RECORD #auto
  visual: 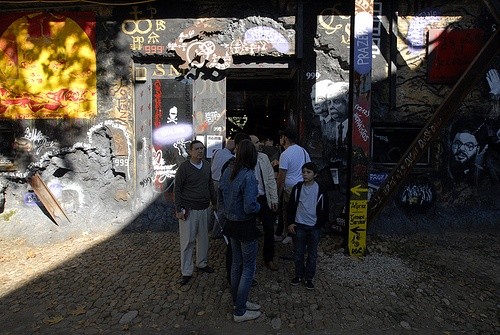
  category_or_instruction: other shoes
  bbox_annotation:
[281,236,293,245]
[196,265,213,273]
[304,279,314,290]
[181,276,190,285]
[233,301,262,311]
[290,276,304,285]
[273,235,283,241]
[233,310,261,323]
[266,261,278,270]
[251,279,257,286]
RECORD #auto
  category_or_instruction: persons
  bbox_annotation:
[310,79,336,149]
[326,81,348,145]
[219,139,261,322]
[209,135,279,285]
[274,128,311,244]
[173,141,216,284]
[285,163,326,290]
